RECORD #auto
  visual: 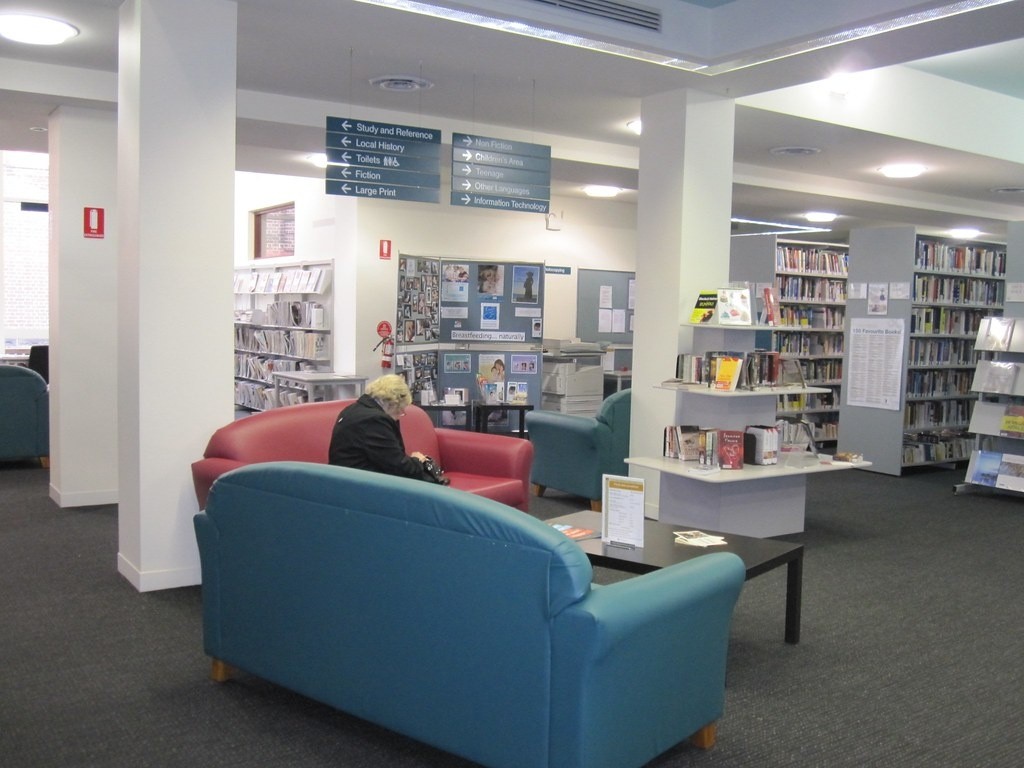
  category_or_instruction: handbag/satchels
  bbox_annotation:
[420,455,451,486]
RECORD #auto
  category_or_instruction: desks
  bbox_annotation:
[474,399,535,439]
[271,371,369,408]
[605,370,631,391]
[419,401,473,432]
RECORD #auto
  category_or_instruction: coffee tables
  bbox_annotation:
[543,509,806,646]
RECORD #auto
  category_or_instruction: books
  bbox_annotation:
[972,450,1003,486]
[1000,395,1024,439]
[833,451,863,462]
[902,240,1006,465]
[775,246,848,456]
[662,286,781,470]
[549,523,601,541]
[995,453,1024,493]
[235,270,328,409]
[983,362,1017,394]
[983,318,1014,350]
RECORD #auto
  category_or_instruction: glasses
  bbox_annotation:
[400,408,407,419]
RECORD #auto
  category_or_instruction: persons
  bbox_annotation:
[397,258,440,341]
[447,361,469,370]
[509,386,515,394]
[521,363,534,370]
[489,392,496,401]
[444,265,468,281]
[490,360,505,382]
[330,374,438,482]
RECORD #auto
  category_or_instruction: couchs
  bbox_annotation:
[0,364,50,468]
[191,398,534,515]
[523,386,631,512]
[193,460,746,768]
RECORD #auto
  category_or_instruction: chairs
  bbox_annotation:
[28,345,49,383]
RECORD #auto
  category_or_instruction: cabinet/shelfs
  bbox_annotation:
[724,233,849,447]
[837,225,1006,477]
[622,323,873,539]
[234,258,336,415]
